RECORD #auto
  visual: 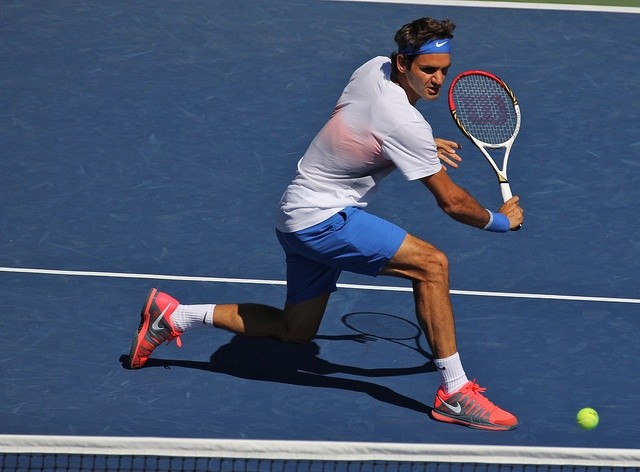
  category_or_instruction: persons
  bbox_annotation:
[130,16,523,431]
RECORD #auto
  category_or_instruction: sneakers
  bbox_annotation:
[431,378,518,431]
[128,287,184,369]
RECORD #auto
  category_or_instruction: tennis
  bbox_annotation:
[575,407,600,430]
